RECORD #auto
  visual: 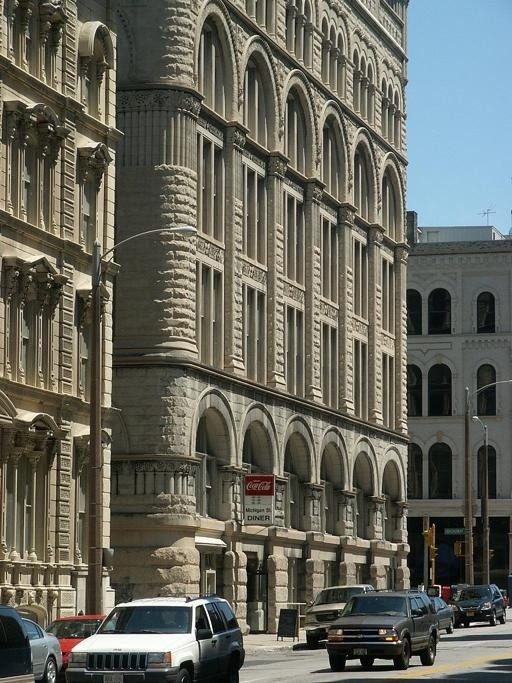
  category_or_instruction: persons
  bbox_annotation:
[69,623,77,634]
[174,611,187,627]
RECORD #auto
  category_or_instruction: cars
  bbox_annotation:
[0,606,111,683]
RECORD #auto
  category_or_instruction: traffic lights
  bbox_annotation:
[429,546,439,559]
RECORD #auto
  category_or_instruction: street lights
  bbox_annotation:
[85,227,200,606]
[472,414,491,585]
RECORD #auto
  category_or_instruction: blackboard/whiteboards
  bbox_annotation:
[278,609,298,637]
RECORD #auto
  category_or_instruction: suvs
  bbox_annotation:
[305,585,455,669]
[454,584,506,626]
[65,594,245,682]
